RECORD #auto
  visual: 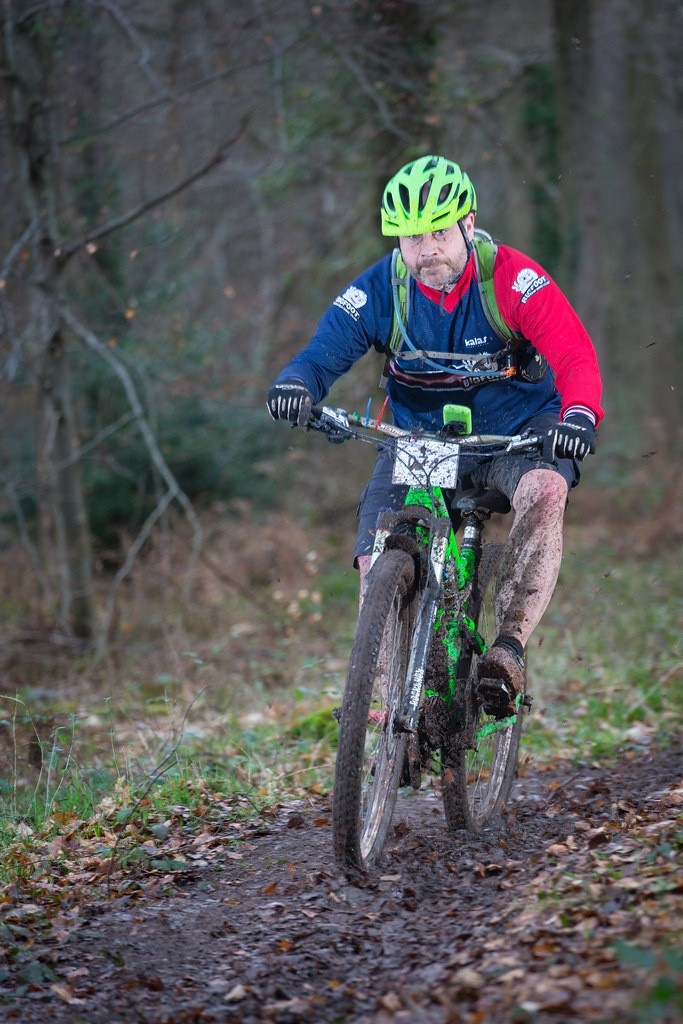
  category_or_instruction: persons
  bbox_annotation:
[265,156,604,784]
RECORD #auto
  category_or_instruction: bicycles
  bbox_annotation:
[289,402,599,884]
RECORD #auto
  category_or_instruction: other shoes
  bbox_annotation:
[370,734,431,777]
[477,644,524,716]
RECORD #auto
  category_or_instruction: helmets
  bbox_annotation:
[381,155,477,237]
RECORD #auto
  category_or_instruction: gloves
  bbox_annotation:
[266,380,315,433]
[543,412,596,464]
